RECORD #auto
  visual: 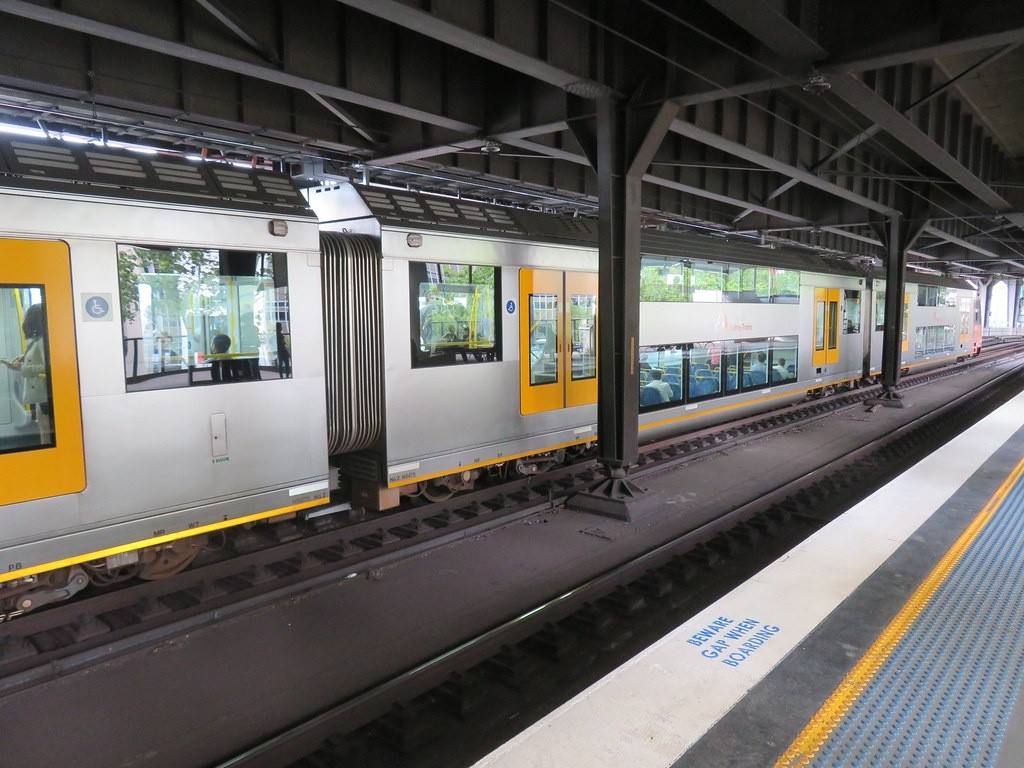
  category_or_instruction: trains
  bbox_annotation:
[0,97,983,590]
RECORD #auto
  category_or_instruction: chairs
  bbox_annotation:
[639,362,795,406]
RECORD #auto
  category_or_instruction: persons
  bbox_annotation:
[442,326,483,360]
[640,354,652,372]
[420,286,474,351]
[646,371,673,403]
[707,343,731,374]
[202,335,232,363]
[154,333,181,370]
[751,353,767,373]
[773,358,789,380]
[11,303,51,445]
[270,321,290,379]
[546,323,555,362]
